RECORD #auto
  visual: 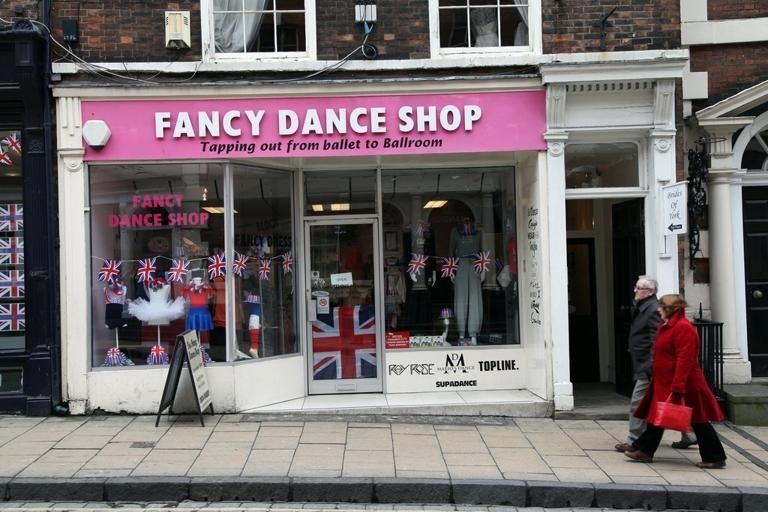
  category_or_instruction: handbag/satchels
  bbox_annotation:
[652,389,693,432]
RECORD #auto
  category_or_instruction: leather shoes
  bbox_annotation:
[696,460,726,468]
[625,449,654,463]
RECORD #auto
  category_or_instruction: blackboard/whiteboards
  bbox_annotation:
[183,329,212,414]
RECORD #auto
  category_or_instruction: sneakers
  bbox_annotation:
[671,440,697,450]
[615,442,634,451]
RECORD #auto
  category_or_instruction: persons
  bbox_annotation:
[615,275,698,454]
[247,302,259,359]
[182,274,215,333]
[623,293,727,469]
[103,283,130,329]
[445,209,485,346]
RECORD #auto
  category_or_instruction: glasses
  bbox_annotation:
[635,285,652,291]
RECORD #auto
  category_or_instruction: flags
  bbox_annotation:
[472,249,491,274]
[0,302,25,332]
[96,259,121,284]
[405,254,429,277]
[1,132,22,158]
[0,237,24,264]
[439,257,459,279]
[0,147,16,168]
[0,203,24,232]
[134,250,294,283]
[0,270,24,299]
[311,304,376,380]
